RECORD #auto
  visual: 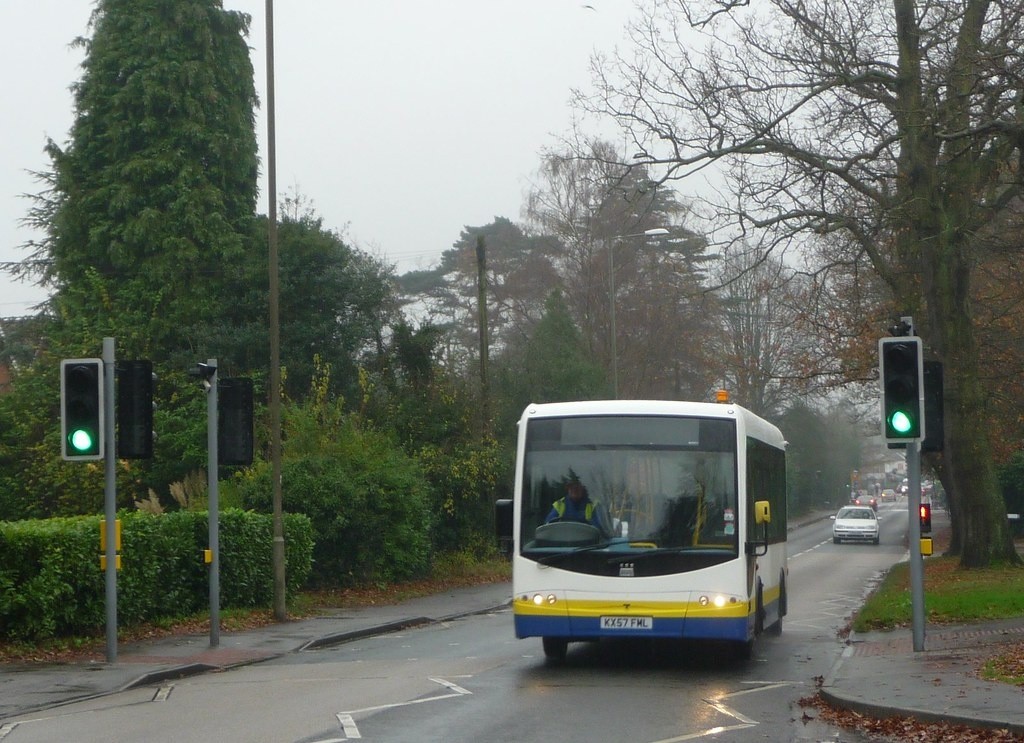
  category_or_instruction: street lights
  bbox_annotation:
[607,228,669,399]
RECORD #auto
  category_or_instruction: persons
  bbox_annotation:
[544,479,602,528]
[851,488,862,502]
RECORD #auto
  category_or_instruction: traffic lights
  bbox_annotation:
[878,336,926,444]
[61,358,105,460]
[920,503,931,533]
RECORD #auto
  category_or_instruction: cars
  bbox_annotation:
[855,495,878,510]
[882,489,896,501]
[830,506,882,544]
[921,480,936,495]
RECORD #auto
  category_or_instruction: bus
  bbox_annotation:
[496,389,789,661]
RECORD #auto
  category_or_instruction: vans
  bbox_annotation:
[901,478,909,495]
